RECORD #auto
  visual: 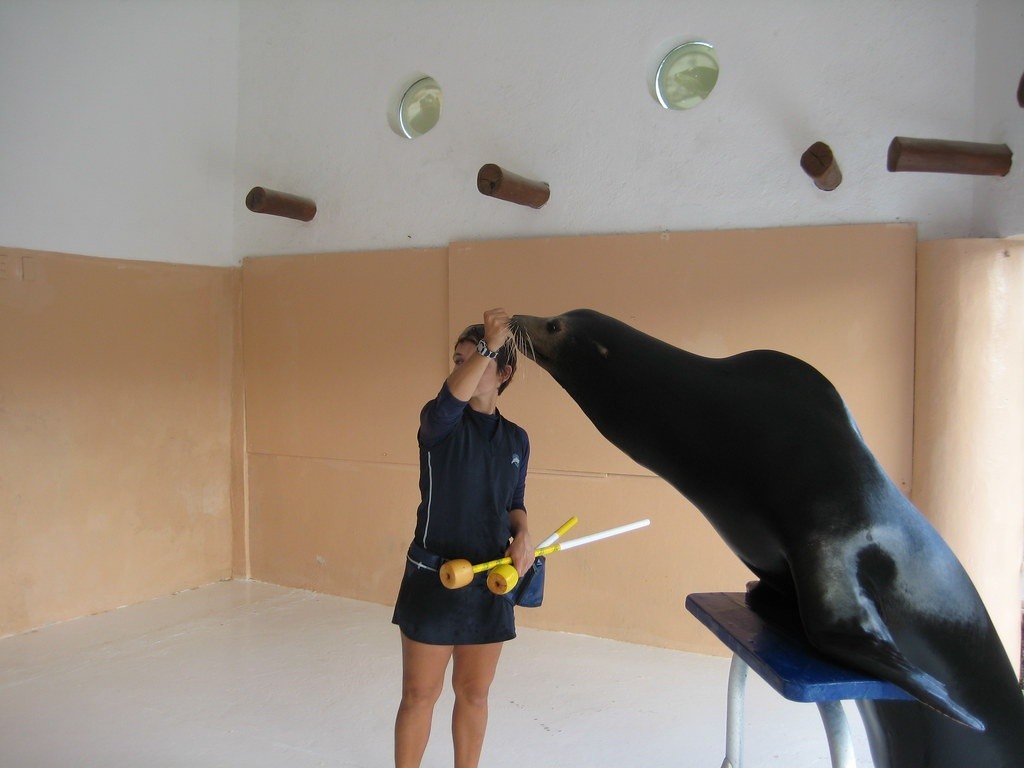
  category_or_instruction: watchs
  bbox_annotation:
[476,339,498,359]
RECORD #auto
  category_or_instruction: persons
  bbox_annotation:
[391,305,535,768]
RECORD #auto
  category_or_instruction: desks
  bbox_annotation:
[684,590,914,768]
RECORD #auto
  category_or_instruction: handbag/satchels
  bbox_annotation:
[514,554,546,608]
